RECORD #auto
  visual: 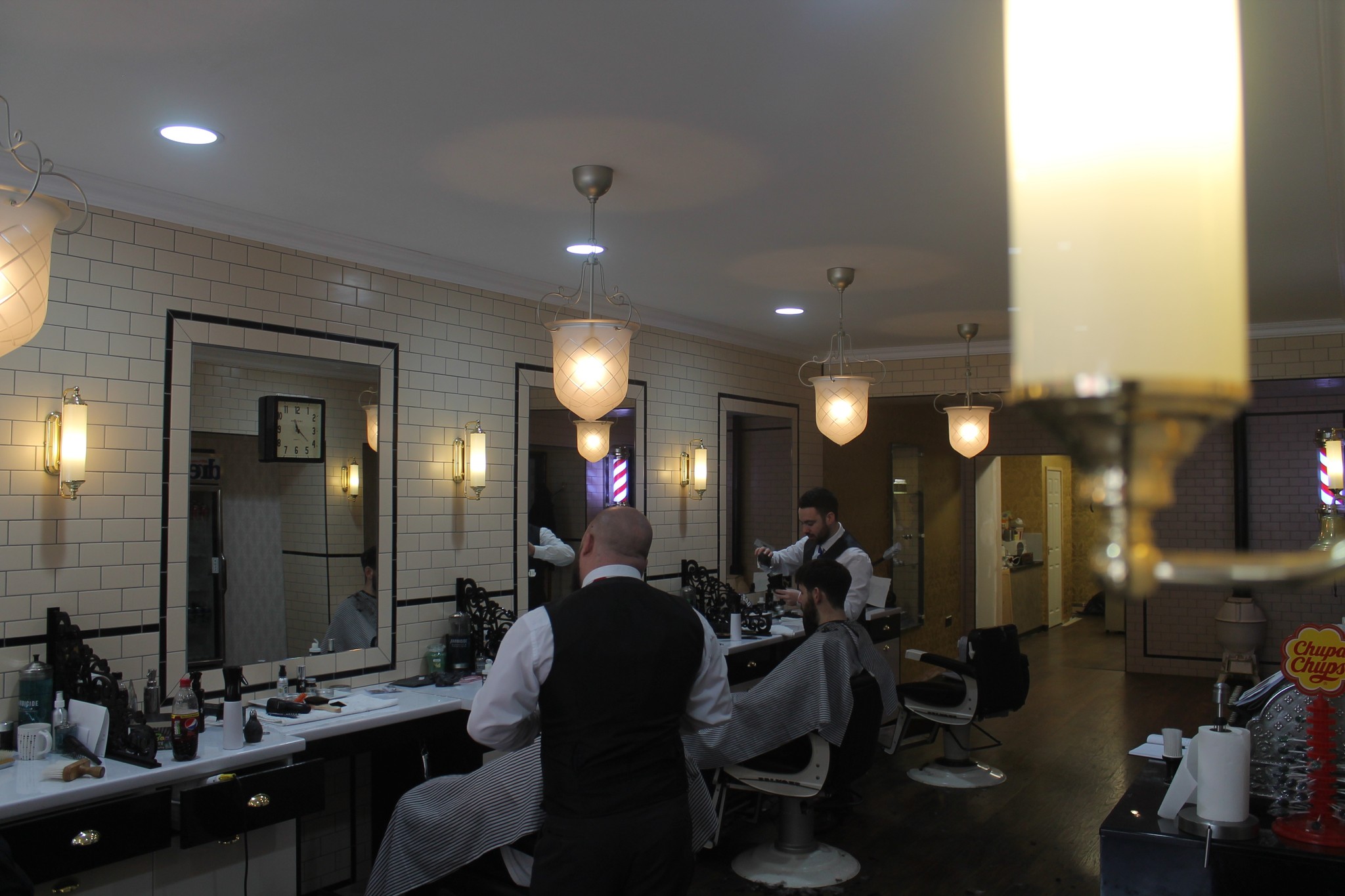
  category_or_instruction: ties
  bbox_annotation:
[814,546,825,559]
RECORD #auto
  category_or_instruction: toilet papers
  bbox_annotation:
[1157,725,1253,821]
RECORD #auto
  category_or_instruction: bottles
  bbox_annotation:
[424,642,447,674]
[1001,545,1006,566]
[482,659,493,686]
[54,719,77,753]
[475,653,486,675]
[171,678,199,761]
[296,665,305,693]
[680,583,696,609]
[449,611,472,635]
[277,665,289,696]
[19,654,54,724]
[112,672,129,736]
[757,597,765,612]
[309,639,321,656]
[52,691,68,753]
[244,709,263,743]
[306,678,317,693]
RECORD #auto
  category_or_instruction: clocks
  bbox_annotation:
[265,395,325,464]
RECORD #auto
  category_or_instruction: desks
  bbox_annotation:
[1099,758,1345,896]
[1002,562,1043,637]
[246,683,462,896]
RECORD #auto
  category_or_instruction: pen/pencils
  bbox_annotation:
[1182,746,1188,749]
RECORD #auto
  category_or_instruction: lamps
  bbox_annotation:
[798,266,886,448]
[536,165,642,422]
[342,456,360,502]
[612,448,627,502]
[0,93,88,358]
[679,438,708,501]
[452,420,486,501]
[933,323,1004,460]
[568,411,618,464]
[359,387,378,453]
[1000,0,1344,598]
[45,386,87,501]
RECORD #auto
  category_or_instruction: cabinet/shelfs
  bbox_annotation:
[724,613,901,721]
[0,756,325,896]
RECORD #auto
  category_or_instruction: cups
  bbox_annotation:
[1014,535,1019,541]
[1004,530,1015,542]
[18,723,52,760]
[1002,556,1013,568]
[0,721,14,750]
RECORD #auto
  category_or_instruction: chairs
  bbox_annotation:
[703,669,883,889]
[882,625,1030,789]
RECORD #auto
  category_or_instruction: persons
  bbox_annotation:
[364,729,547,896]
[317,545,378,655]
[466,506,735,896]
[755,485,873,636]
[679,558,873,855]
[529,510,576,609]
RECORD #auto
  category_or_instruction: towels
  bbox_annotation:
[769,625,796,638]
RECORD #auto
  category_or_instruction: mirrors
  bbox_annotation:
[725,410,794,595]
[527,385,637,610]
[186,341,381,672]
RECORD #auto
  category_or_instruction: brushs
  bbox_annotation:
[42,758,105,781]
[0,750,15,769]
[1162,728,1183,785]
[292,692,306,703]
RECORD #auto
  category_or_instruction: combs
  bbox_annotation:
[62,735,103,767]
